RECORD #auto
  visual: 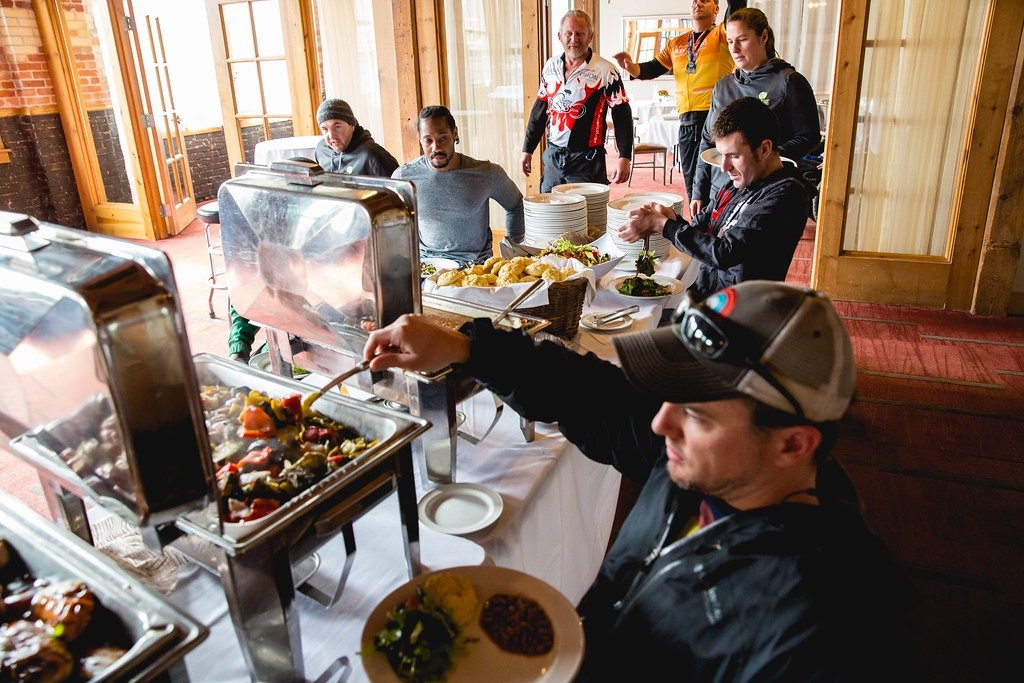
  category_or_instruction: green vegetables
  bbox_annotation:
[371,587,464,683]
[618,251,673,296]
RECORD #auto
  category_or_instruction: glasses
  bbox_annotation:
[671,289,808,417]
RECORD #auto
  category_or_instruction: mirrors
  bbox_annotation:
[620,12,695,80]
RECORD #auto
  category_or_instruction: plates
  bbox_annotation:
[412,534,486,573]
[582,310,633,330]
[700,146,797,173]
[417,480,504,534]
[520,180,684,270]
[359,563,585,682]
[606,274,685,299]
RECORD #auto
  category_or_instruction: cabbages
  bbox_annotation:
[420,260,435,276]
[528,239,613,267]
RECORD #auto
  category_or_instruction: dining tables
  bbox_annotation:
[77,231,702,683]
[641,111,682,184]
[636,93,678,125]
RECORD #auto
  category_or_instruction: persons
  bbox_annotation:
[618,95,808,313]
[517,10,635,193]
[686,7,818,217]
[311,97,398,180]
[391,105,525,270]
[228,307,257,364]
[360,280,894,683]
[612,0,750,222]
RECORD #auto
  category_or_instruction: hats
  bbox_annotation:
[614,280,854,423]
[316,98,360,127]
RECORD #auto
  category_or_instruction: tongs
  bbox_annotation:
[593,304,640,326]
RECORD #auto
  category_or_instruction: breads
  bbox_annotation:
[423,574,479,627]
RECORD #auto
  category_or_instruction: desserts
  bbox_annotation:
[437,255,577,287]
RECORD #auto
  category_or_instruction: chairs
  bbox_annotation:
[625,116,668,185]
[605,122,619,153]
